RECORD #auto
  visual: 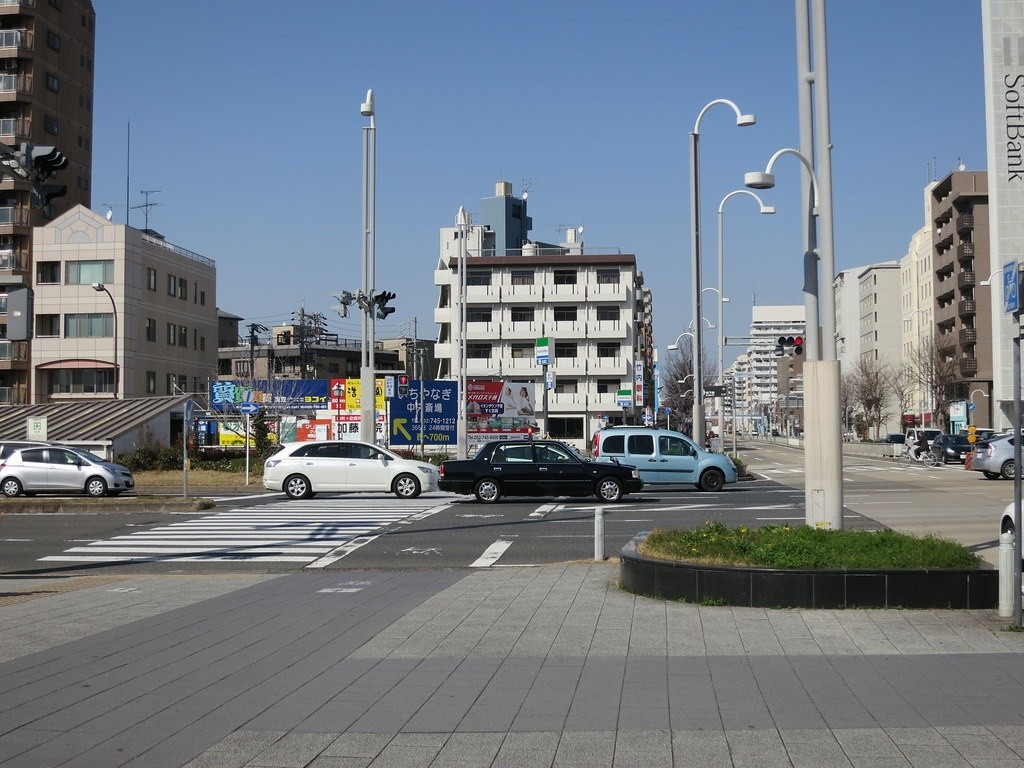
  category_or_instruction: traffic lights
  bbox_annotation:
[793,345,803,355]
[774,336,803,347]
[397,375,408,396]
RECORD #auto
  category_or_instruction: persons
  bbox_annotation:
[539,446,551,463]
[912,431,929,459]
[516,387,535,416]
[660,442,669,454]
[502,386,517,409]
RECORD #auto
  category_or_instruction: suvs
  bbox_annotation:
[0,440,82,474]
[262,441,440,499]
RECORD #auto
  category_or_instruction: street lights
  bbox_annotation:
[91,282,119,399]
[743,147,847,530]
[716,186,777,451]
[665,285,732,443]
[684,96,756,446]
[970,388,990,428]
[356,87,380,443]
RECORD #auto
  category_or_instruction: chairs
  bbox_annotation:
[496,448,541,463]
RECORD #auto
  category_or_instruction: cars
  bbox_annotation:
[437,439,643,503]
[997,497,1024,561]
[0,446,135,498]
[589,426,738,492]
[880,422,1023,481]
